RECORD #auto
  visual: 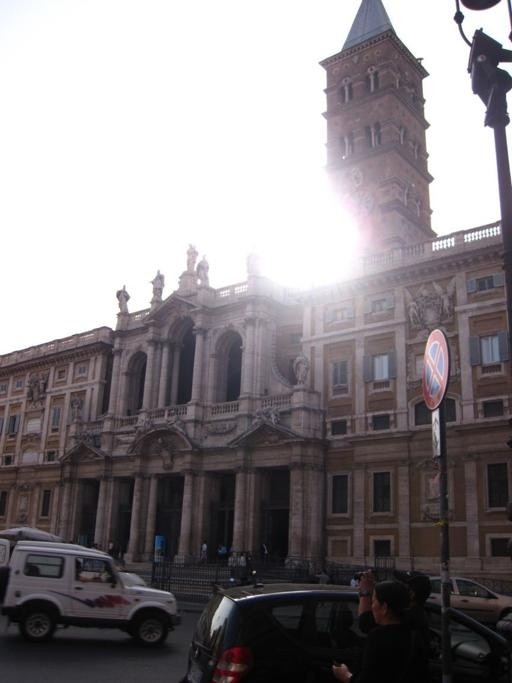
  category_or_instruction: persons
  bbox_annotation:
[91,538,125,568]
[358,569,433,683]
[198,539,272,587]
[315,569,331,584]
[351,574,359,587]
[331,582,421,682]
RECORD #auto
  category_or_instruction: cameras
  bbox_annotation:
[353,571,368,582]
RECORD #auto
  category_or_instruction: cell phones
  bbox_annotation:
[333,661,342,667]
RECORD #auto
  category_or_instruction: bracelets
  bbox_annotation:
[359,589,369,598]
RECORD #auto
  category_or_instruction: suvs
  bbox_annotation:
[0,526,183,646]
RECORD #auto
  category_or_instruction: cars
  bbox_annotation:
[179,578,512,682]
[425,575,512,631]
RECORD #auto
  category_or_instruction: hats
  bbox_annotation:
[392,568,432,601]
